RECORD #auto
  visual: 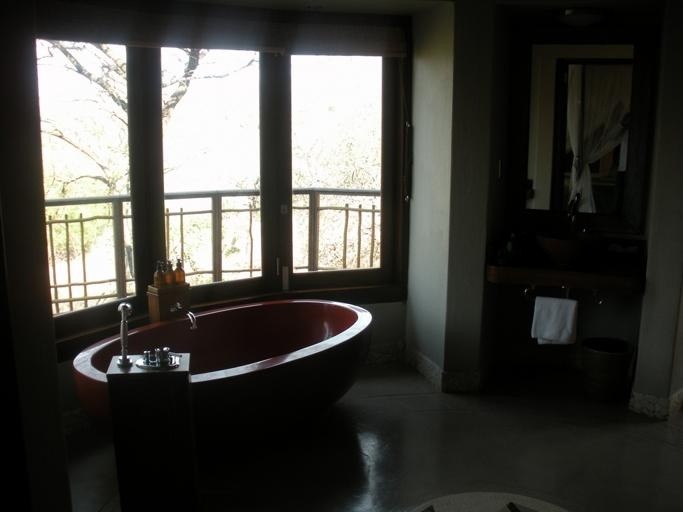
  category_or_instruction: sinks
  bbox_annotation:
[536,227,594,258]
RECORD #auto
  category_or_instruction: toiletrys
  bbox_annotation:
[153,260,185,285]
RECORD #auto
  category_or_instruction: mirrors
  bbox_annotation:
[504,22,659,241]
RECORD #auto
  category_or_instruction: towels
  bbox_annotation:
[530,295,578,345]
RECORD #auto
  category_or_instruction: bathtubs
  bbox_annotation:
[72,299,374,443]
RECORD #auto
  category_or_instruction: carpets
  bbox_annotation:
[380,480,585,512]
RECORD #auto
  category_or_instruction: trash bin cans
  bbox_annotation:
[577,335,634,413]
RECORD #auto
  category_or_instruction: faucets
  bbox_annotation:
[116,301,132,365]
[565,193,579,227]
